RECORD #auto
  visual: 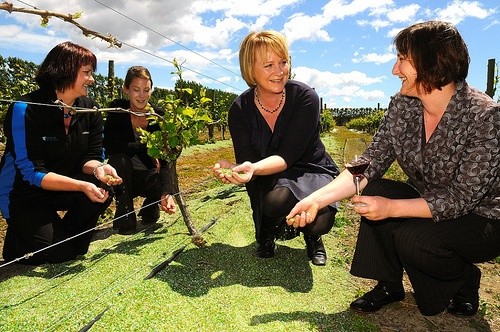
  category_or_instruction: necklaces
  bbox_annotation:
[255,87,284,113]
[64,113,69,119]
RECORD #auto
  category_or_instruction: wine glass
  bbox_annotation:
[342,137,370,206]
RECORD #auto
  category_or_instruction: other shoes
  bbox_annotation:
[113,198,137,235]
[138,199,160,224]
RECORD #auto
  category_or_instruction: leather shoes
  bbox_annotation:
[303,232,327,266]
[447,262,482,317]
[350,277,405,313]
[255,234,276,258]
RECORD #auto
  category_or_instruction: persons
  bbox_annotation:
[0,41,123,265]
[285,21,500,316]
[103,67,177,236]
[213,30,340,266]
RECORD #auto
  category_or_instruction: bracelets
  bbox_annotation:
[93,163,106,181]
